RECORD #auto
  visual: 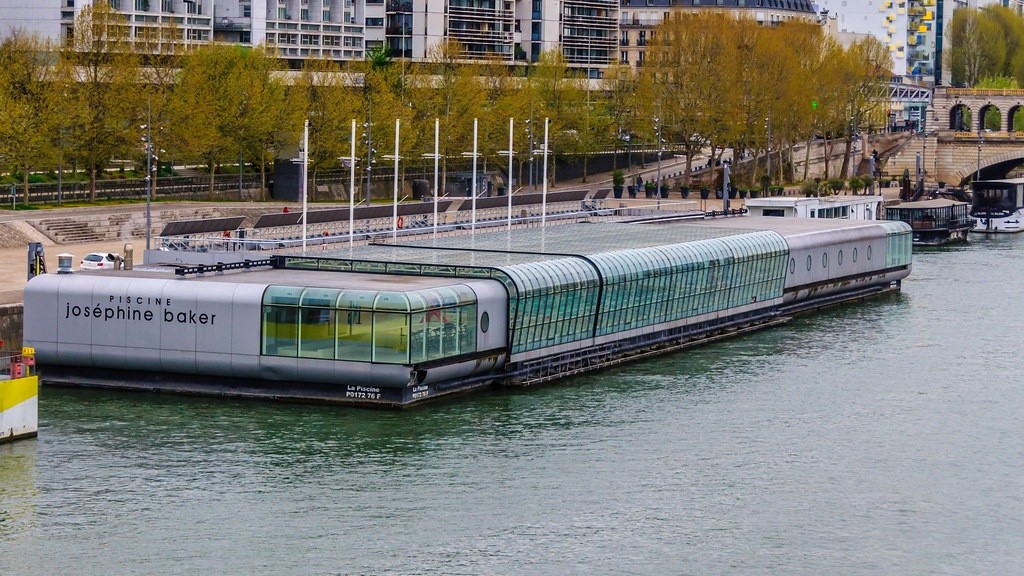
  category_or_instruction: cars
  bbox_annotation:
[81,252,125,270]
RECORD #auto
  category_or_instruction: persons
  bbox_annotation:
[871,149,879,164]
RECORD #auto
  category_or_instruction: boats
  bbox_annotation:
[885,198,977,248]
[743,194,883,221]
[968,179,1024,232]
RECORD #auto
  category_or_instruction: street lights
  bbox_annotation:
[976,111,985,181]
[363,98,378,205]
[525,102,538,194]
[653,100,666,211]
[764,102,774,195]
[851,102,859,176]
[140,98,168,248]
[921,108,927,187]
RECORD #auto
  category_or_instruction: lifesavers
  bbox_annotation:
[397,216,403,230]
